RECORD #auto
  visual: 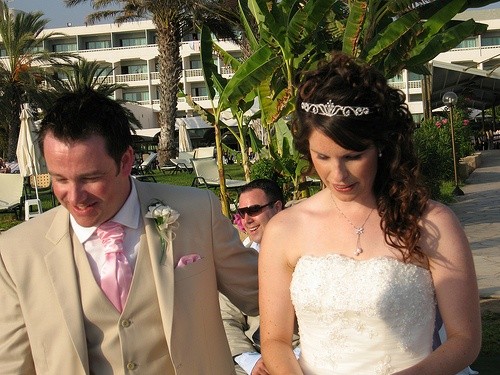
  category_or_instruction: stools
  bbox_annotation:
[24,198,43,222]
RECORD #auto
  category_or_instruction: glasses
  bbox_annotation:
[237,200,275,219]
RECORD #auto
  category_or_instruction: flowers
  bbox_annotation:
[145,196,180,264]
[233,212,247,232]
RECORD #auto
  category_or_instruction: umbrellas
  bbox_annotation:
[16,109,49,199]
[178,120,193,152]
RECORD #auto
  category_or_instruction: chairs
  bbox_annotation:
[130,146,247,194]
[0,171,24,222]
[25,173,54,207]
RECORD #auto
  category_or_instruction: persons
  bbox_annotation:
[219,180,301,375]
[258,53,482,375]
[0,88,260,375]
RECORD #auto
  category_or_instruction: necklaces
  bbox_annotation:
[332,192,376,256]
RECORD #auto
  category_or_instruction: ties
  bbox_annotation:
[96,220,133,314]
